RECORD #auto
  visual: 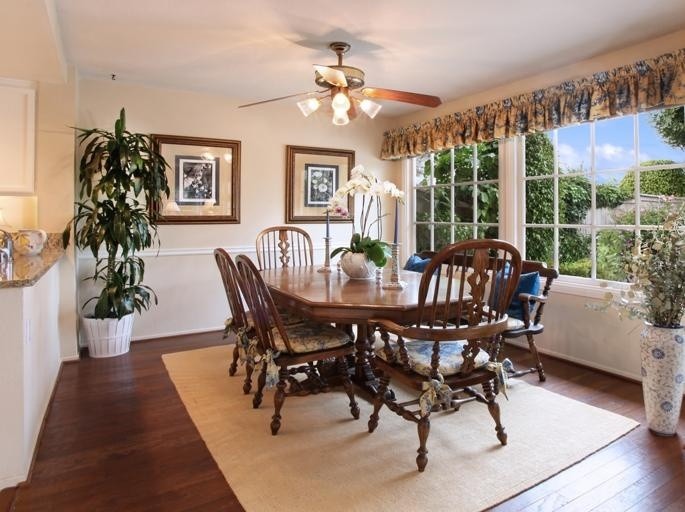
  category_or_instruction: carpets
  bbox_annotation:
[159,342,643,512]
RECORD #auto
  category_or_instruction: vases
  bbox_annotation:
[338,249,379,283]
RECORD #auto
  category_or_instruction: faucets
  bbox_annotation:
[0,229,14,261]
[0,261,14,280]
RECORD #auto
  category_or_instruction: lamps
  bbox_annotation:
[295,86,382,127]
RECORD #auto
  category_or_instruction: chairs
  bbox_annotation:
[212,247,307,395]
[254,227,314,271]
[234,253,361,436]
[363,237,523,474]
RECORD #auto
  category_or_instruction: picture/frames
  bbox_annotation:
[285,144,356,224]
[149,133,242,226]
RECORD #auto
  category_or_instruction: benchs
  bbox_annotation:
[405,248,560,385]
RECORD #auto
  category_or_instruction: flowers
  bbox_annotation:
[326,163,407,270]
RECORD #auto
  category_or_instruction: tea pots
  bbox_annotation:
[11,228,47,256]
[14,256,44,277]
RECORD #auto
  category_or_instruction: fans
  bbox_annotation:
[237,41,443,120]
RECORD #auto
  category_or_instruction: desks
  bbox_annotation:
[247,263,474,403]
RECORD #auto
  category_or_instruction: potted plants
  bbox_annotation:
[585,193,685,440]
[61,106,172,360]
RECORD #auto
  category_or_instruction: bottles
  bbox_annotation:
[336,260,342,273]
[375,268,383,285]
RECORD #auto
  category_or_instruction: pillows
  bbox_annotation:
[407,253,439,276]
[491,259,542,327]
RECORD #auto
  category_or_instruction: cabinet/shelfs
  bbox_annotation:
[0,75,37,197]
